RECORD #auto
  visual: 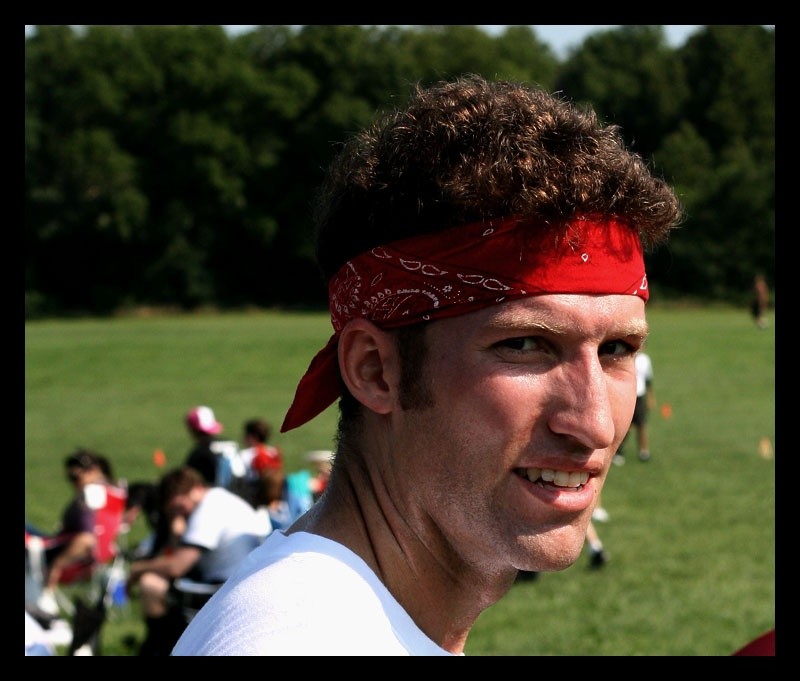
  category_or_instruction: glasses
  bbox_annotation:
[70,472,81,483]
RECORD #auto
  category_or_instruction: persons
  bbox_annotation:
[172,71,774,655]
[611,352,655,466]
[750,273,769,330]
[25,405,336,656]
[517,519,606,580]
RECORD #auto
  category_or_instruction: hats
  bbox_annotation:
[186,406,222,434]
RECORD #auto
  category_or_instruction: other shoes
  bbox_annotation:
[588,544,607,568]
[37,588,59,618]
[635,447,650,459]
[613,453,627,464]
[591,507,607,520]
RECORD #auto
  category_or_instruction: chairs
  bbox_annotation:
[23,481,226,658]
